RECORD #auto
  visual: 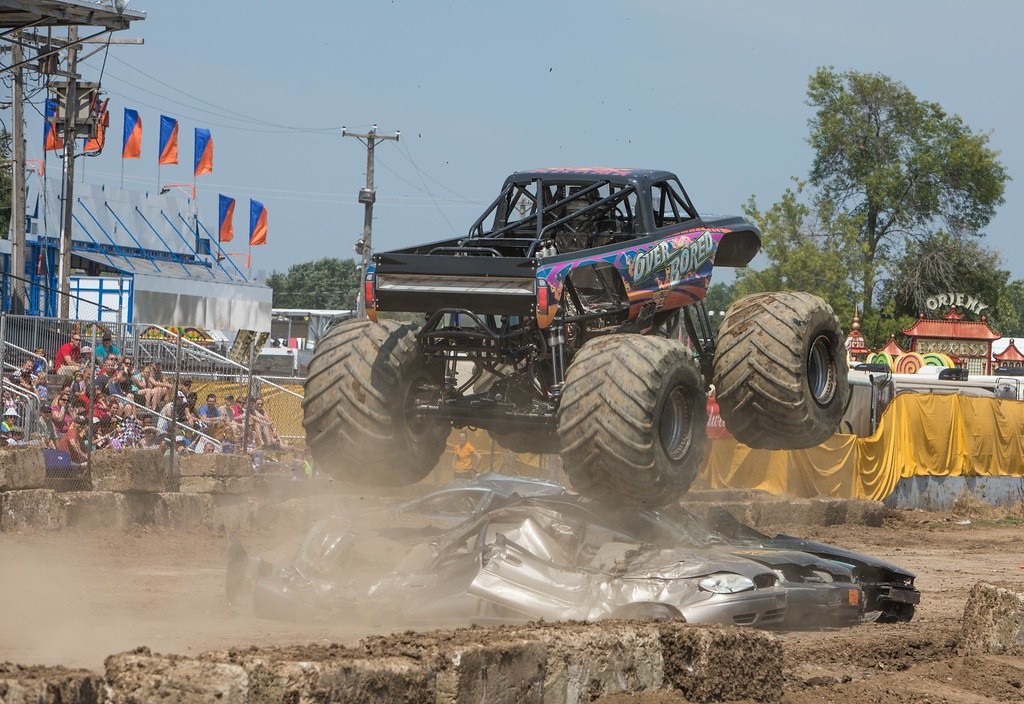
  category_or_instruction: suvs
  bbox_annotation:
[302,167,850,508]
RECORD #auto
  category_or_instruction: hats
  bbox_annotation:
[40,406,52,411]
[176,390,186,398]
[175,436,185,441]
[224,395,234,399]
[21,372,31,378]
[1,407,22,418]
[76,406,87,414]
[182,379,194,384]
[102,334,111,339]
[152,358,162,365]
[142,426,159,433]
[80,346,91,353]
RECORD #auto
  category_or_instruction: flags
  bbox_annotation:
[219,193,235,242]
[42,99,215,176]
[248,199,268,246]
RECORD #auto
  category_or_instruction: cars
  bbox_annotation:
[249,470,922,632]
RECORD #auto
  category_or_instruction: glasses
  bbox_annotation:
[43,410,52,413]
[236,401,245,404]
[6,416,16,419]
[72,337,82,341]
[257,403,263,406]
[249,425,254,427]
[108,358,118,361]
[103,339,111,341]
[61,399,69,402]
[125,362,131,365]
[206,449,214,452]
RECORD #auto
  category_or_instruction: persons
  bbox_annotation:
[1,333,285,471]
